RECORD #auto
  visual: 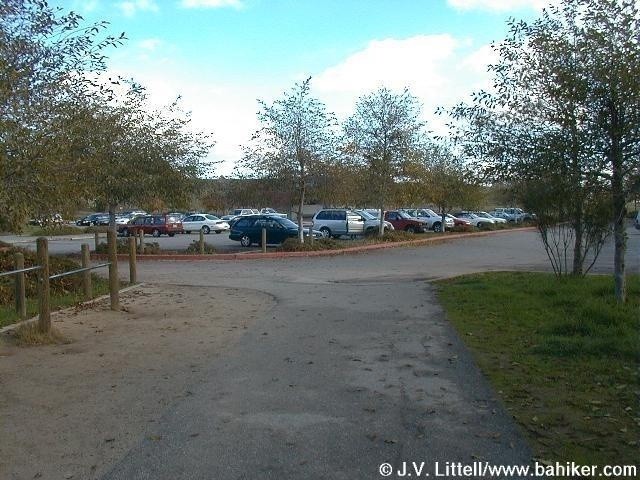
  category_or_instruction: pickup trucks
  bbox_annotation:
[261,207,288,219]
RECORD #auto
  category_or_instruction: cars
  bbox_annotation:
[75,209,150,226]
[180,214,230,235]
[368,208,537,234]
[28,213,64,227]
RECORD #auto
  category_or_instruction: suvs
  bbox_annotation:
[220,209,260,229]
[633,210,640,230]
[311,208,394,239]
[228,213,324,247]
[119,214,184,237]
